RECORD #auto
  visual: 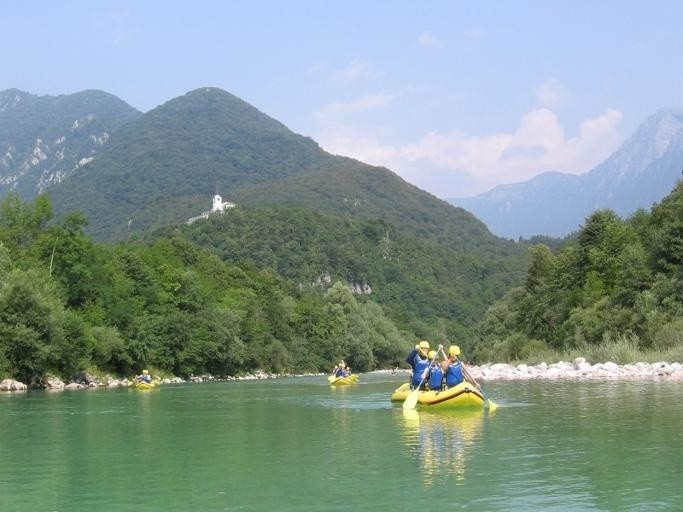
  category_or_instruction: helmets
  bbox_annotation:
[448,345,460,356]
[418,340,429,350]
[334,363,349,370]
[141,369,149,375]
[427,351,438,359]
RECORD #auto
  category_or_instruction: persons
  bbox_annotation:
[442,344,466,387]
[406,340,430,390]
[331,360,353,377]
[423,345,449,391]
[137,370,153,383]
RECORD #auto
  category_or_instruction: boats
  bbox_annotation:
[388,379,491,409]
[325,373,352,386]
[134,378,158,390]
[349,374,358,383]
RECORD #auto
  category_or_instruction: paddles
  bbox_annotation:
[402,346,442,410]
[455,356,498,410]
